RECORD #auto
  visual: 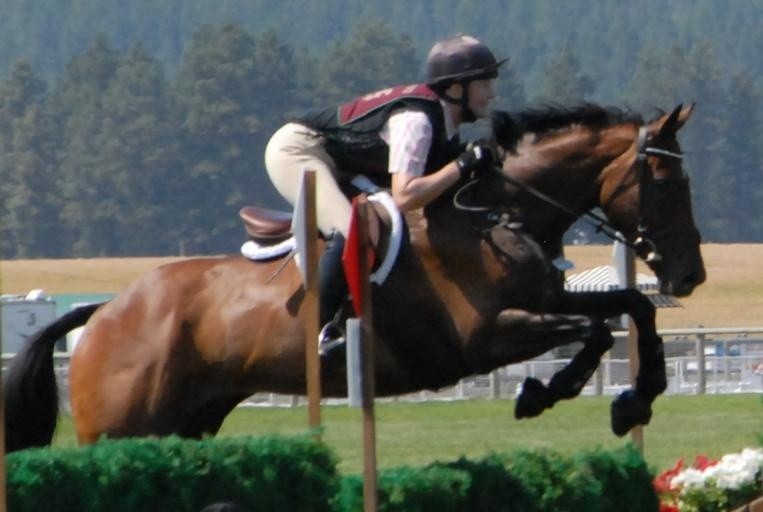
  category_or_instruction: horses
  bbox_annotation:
[0,99,707,454]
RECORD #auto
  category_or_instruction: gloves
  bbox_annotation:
[457,140,490,179]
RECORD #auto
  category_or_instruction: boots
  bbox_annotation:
[317,232,347,344]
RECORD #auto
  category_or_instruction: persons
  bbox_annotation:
[262,35,498,358]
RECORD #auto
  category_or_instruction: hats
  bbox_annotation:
[425,34,510,87]
